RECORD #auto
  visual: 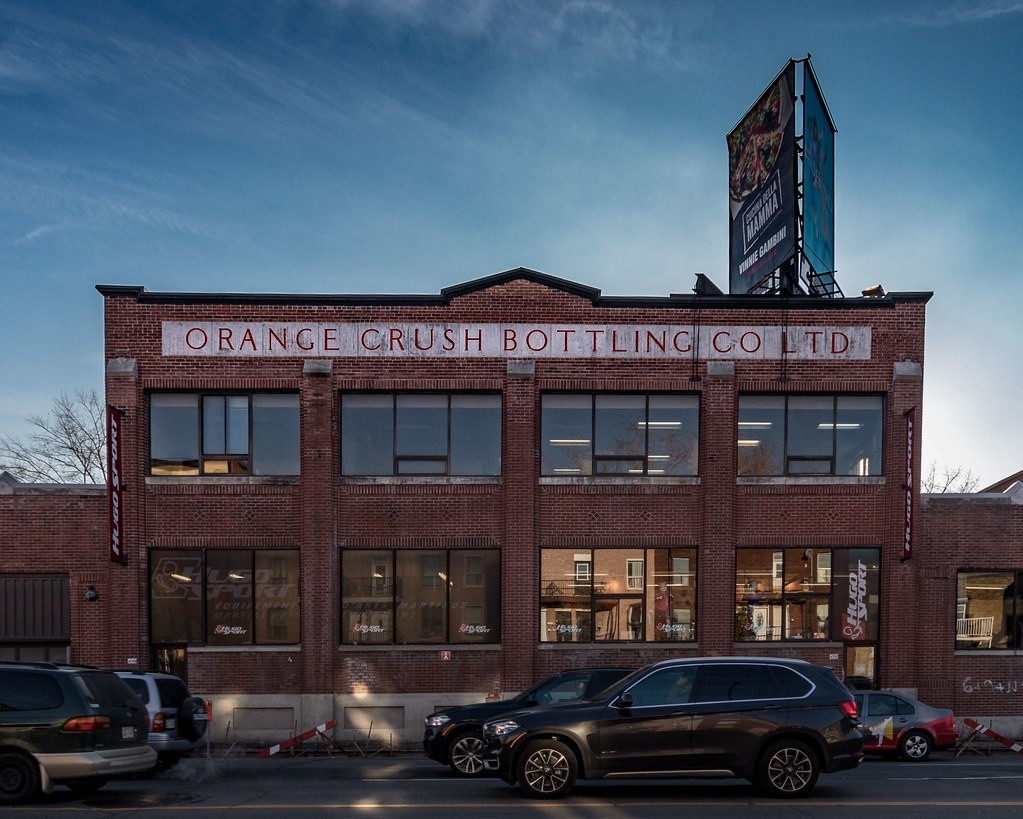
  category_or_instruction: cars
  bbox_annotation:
[850,690,957,764]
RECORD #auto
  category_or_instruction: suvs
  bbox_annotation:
[113,668,209,774]
[480,656,864,801]
[422,668,641,778]
[0,660,159,809]
